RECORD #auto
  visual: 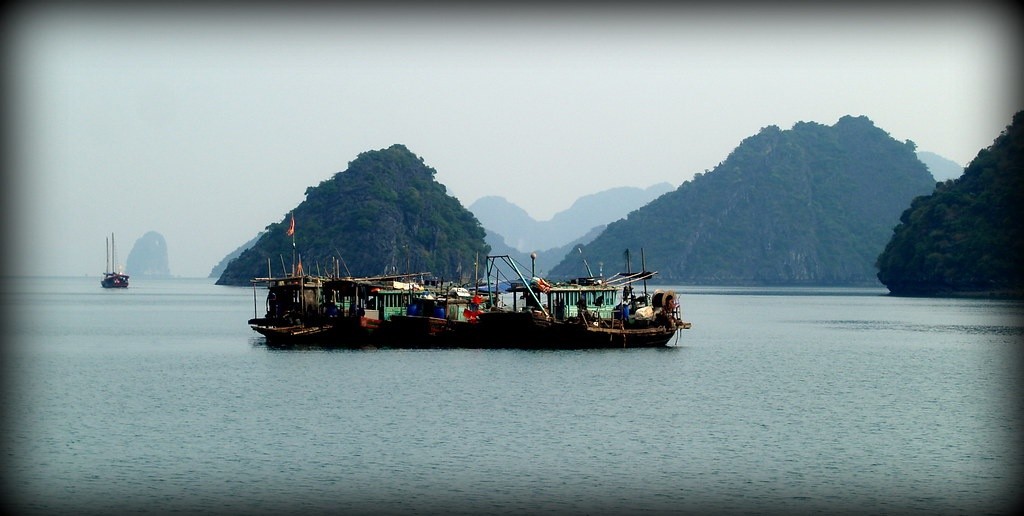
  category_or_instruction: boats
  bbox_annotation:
[101,232,130,289]
[247,213,692,348]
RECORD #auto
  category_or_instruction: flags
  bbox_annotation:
[288,216,296,236]
